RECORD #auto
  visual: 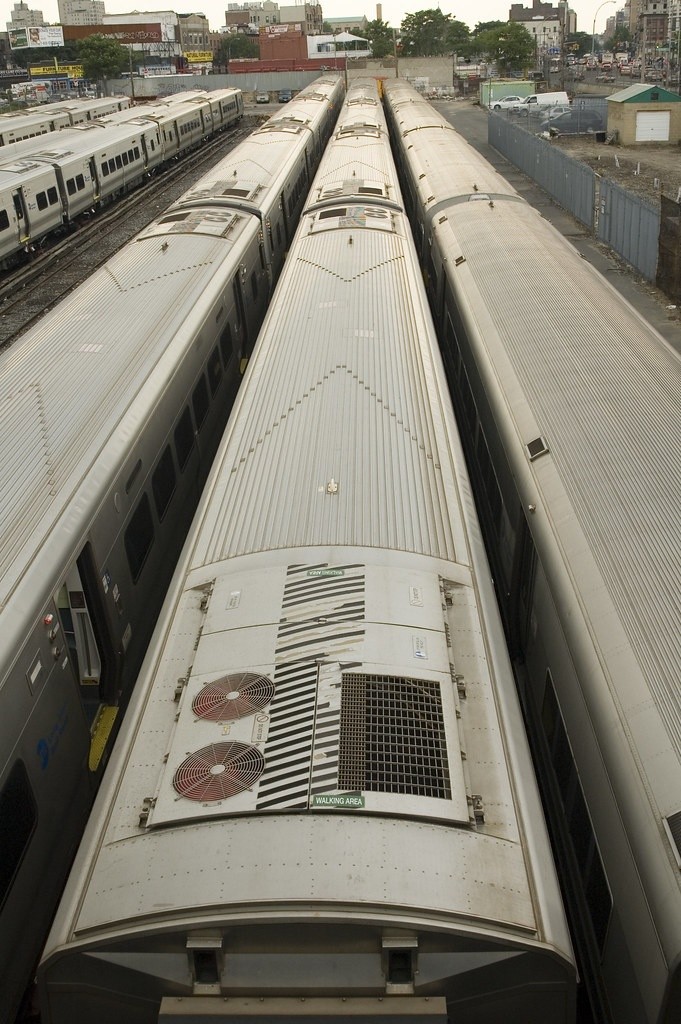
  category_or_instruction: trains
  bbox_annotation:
[0,74,681,1023]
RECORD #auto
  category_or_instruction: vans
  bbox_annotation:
[513,91,570,117]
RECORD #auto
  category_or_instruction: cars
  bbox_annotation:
[541,110,603,133]
[488,96,524,110]
[621,59,681,87]
[539,106,573,121]
[256,92,270,103]
[565,53,628,83]
[278,89,292,103]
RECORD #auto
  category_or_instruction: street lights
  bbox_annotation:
[229,38,240,59]
[591,1,616,72]
[538,15,557,70]
